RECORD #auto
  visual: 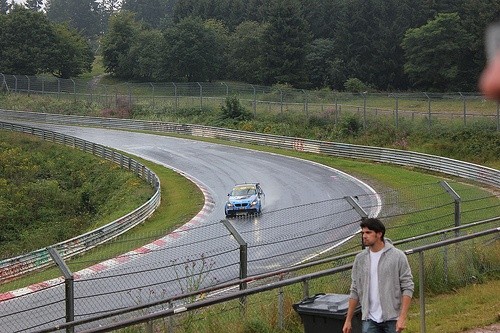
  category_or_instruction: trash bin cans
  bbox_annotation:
[292,290,363,333]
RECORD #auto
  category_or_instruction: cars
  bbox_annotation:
[224,182,266,217]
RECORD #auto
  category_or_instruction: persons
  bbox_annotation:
[342,217,415,333]
